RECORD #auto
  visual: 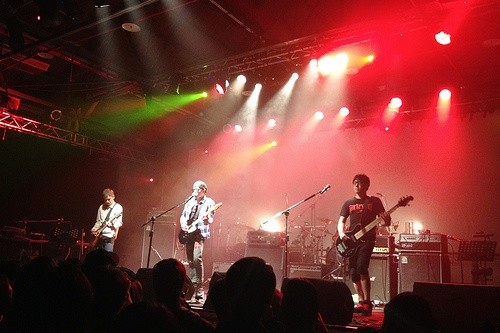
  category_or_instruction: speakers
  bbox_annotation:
[141,221,180,268]
[344,253,397,305]
[289,268,326,279]
[397,253,449,294]
[204,272,226,312]
[244,245,285,293]
[281,278,353,326]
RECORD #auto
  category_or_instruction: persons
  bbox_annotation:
[0,249,499,333]
[180,181,216,303]
[337,174,391,315]
[91,189,123,252]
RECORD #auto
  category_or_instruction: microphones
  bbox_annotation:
[184,192,198,204]
[320,184,330,194]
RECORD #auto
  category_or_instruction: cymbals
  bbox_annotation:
[229,225,256,231]
[314,216,338,225]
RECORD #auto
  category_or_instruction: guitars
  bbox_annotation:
[178,202,223,245]
[335,194,415,258]
[88,211,123,249]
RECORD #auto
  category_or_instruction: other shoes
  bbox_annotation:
[354,301,364,312]
[195,288,205,299]
[362,301,374,316]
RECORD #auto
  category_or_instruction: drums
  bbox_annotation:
[326,246,351,274]
[227,240,246,261]
